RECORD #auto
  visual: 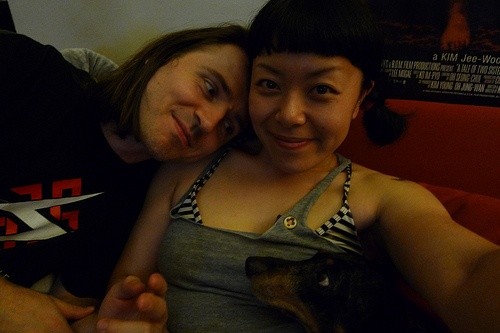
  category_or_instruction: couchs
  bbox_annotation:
[334,98,500,244]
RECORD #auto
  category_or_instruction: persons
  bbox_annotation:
[0,21,259,332]
[105,0,500,333]
